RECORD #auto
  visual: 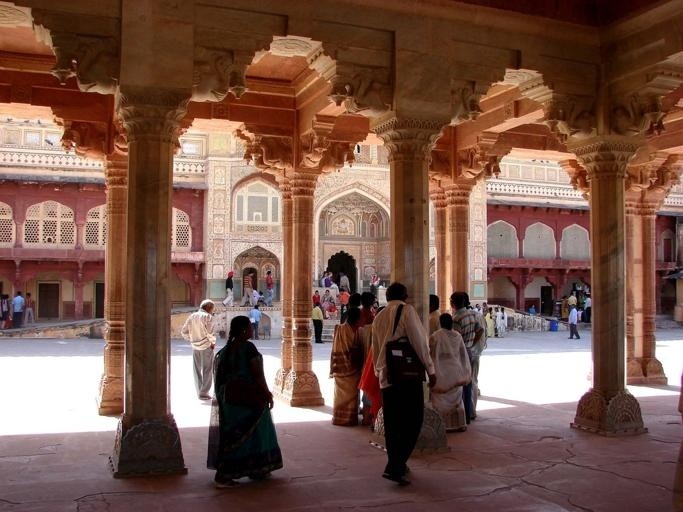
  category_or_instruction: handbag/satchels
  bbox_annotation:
[385,336,427,384]
[250,317,255,323]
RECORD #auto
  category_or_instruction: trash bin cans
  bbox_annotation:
[550,319,558,331]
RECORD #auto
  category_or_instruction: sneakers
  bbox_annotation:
[199,395,211,400]
[212,477,240,488]
[382,464,412,485]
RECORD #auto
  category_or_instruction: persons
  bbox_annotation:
[212,315,283,489]
[371,281,437,486]
[0,293,9,330]
[10,291,25,328]
[464,293,487,421]
[450,291,484,423]
[247,305,262,340]
[181,299,216,401]
[312,270,351,344]
[239,270,256,307]
[222,271,234,307]
[428,292,441,400]
[567,304,580,339]
[562,291,592,323]
[262,270,274,307]
[472,303,507,340]
[23,292,36,324]
[329,291,382,431]
[429,313,472,433]
[528,304,540,317]
